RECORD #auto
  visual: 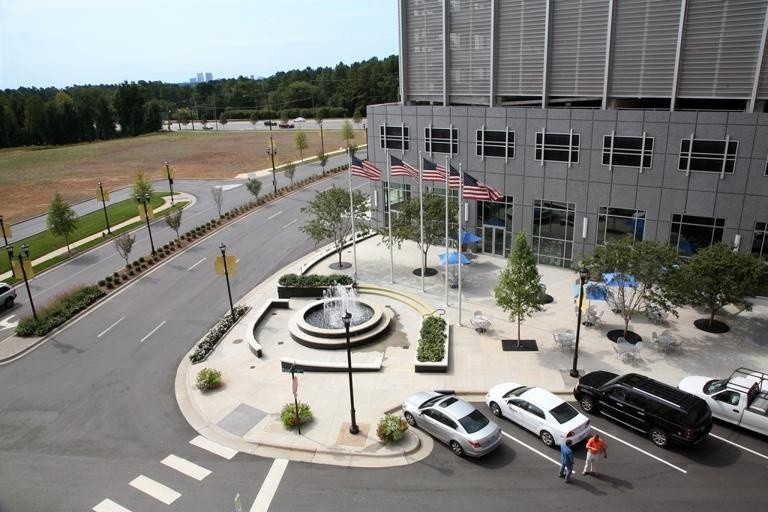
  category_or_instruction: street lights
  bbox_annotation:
[340,308,360,434]
[0,214,37,319]
[319,123,324,154]
[95,161,174,254]
[265,133,277,192]
[570,268,591,377]
[218,242,236,324]
[363,123,369,162]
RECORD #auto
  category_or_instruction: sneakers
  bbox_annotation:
[582,471,596,478]
[558,473,574,484]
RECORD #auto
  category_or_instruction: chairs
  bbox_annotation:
[551,327,577,352]
[469,310,494,334]
[652,331,684,354]
[575,294,669,329]
[612,335,644,365]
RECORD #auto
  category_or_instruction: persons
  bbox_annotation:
[558,434,608,483]
[728,393,740,405]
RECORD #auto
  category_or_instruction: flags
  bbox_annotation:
[350,154,504,202]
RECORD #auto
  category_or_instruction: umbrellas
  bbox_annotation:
[438,229,482,277]
[573,272,639,320]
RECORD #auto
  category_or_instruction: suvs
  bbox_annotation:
[573,369,713,454]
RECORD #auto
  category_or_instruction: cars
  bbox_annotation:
[163,121,173,125]
[402,390,504,458]
[264,117,305,128]
[201,125,213,129]
[678,366,768,438]
[485,382,592,448]
